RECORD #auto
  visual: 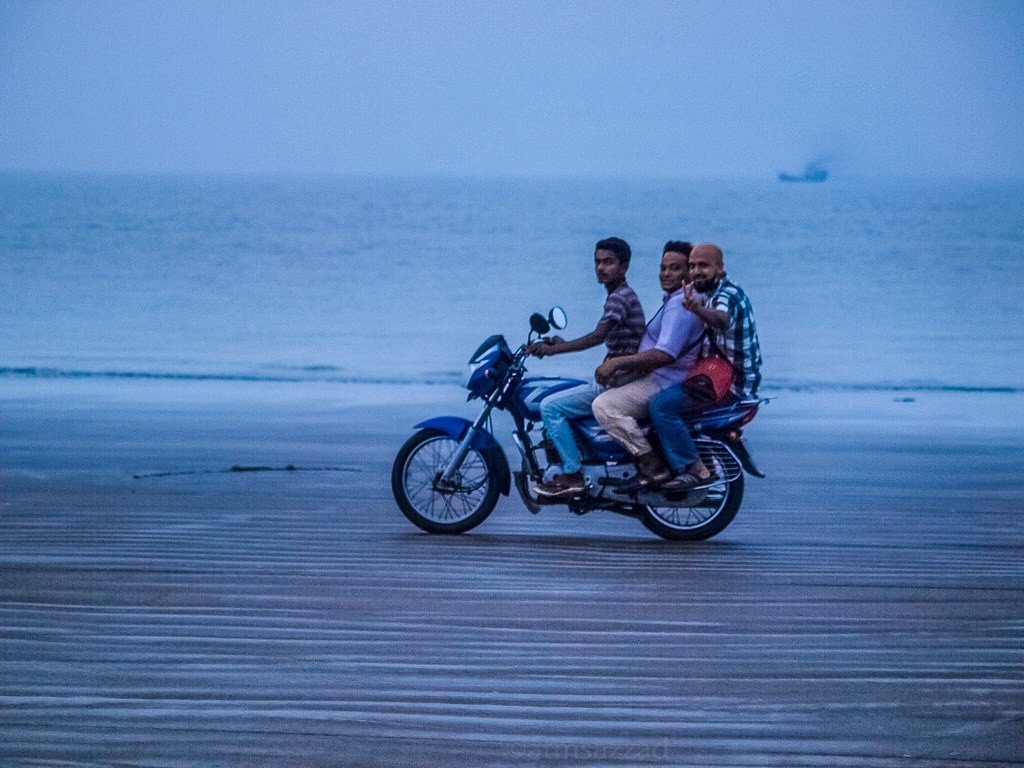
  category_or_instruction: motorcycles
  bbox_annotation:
[390,305,778,542]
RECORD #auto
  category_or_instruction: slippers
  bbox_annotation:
[613,465,672,494]
[532,471,585,496]
[661,470,717,493]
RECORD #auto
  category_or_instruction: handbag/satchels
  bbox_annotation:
[682,357,734,405]
[612,365,645,388]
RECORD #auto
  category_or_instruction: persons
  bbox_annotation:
[525,237,646,497]
[592,240,762,490]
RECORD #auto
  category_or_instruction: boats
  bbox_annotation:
[778,162,832,183]
